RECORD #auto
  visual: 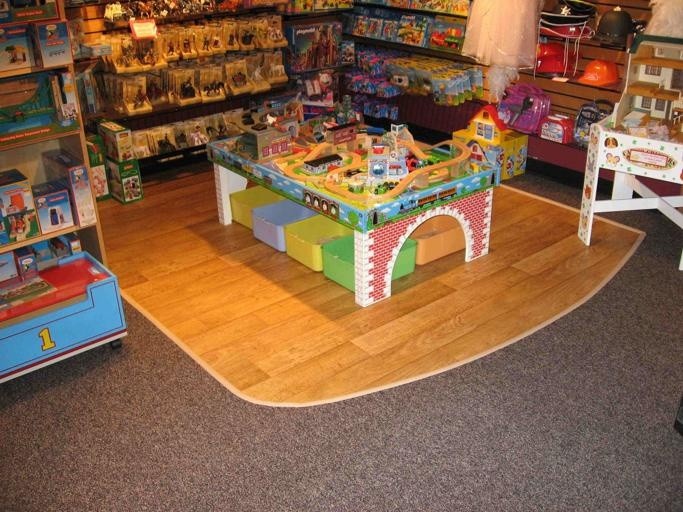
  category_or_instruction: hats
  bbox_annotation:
[539,0,599,39]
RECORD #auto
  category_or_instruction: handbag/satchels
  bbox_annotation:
[573,99,614,150]
[538,111,574,145]
[496,81,550,134]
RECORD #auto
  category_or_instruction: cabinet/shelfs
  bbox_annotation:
[0,1,129,389]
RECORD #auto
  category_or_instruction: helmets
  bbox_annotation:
[535,43,573,74]
[594,6,633,47]
[577,58,617,87]
